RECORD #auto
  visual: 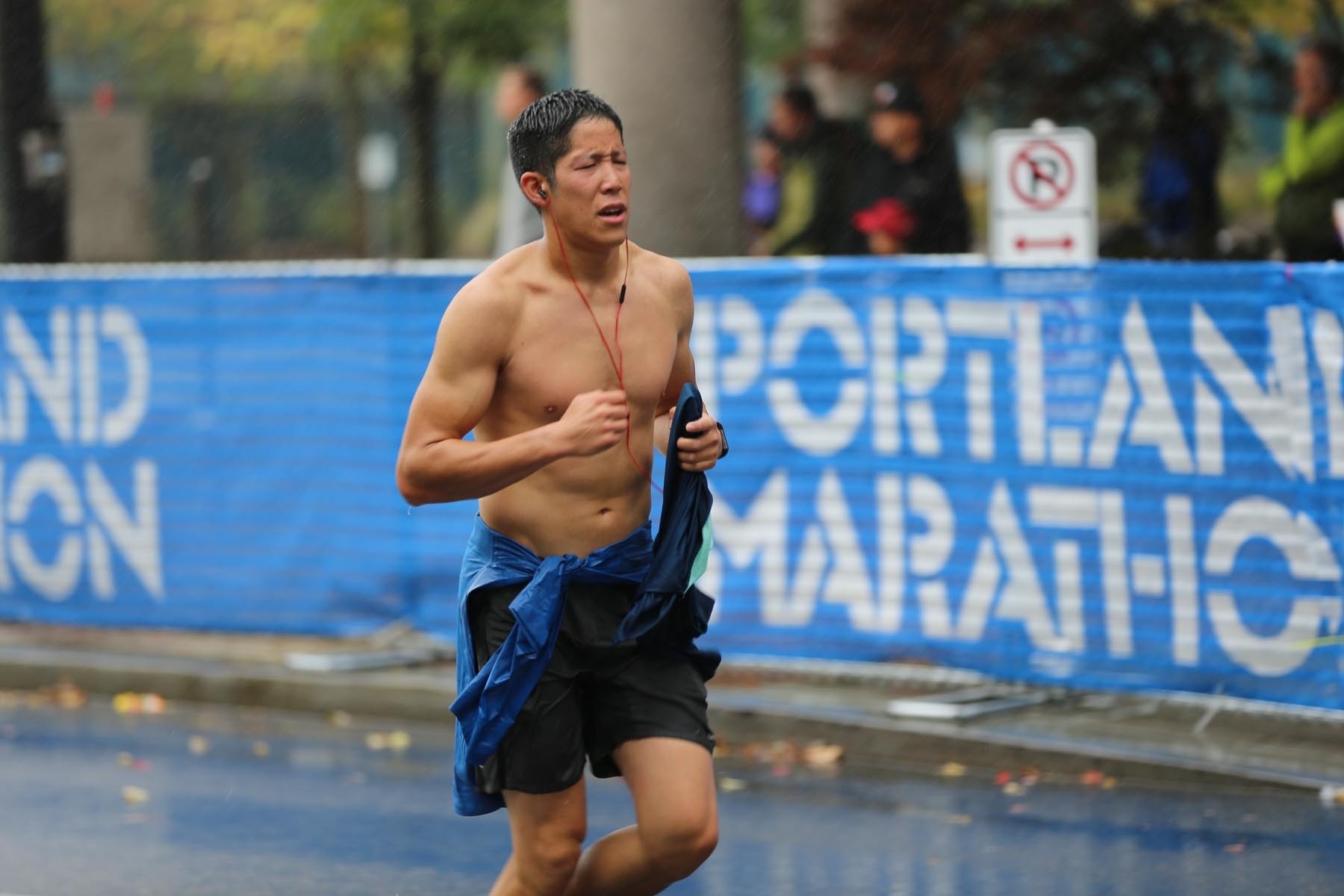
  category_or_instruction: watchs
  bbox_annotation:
[717,421,729,460]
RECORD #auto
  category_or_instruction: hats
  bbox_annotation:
[867,72,920,113]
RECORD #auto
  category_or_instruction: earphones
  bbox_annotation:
[538,188,547,198]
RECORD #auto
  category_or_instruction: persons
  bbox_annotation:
[397,87,724,896]
[492,64,547,262]
[1118,37,1344,261]
[743,76,972,260]
